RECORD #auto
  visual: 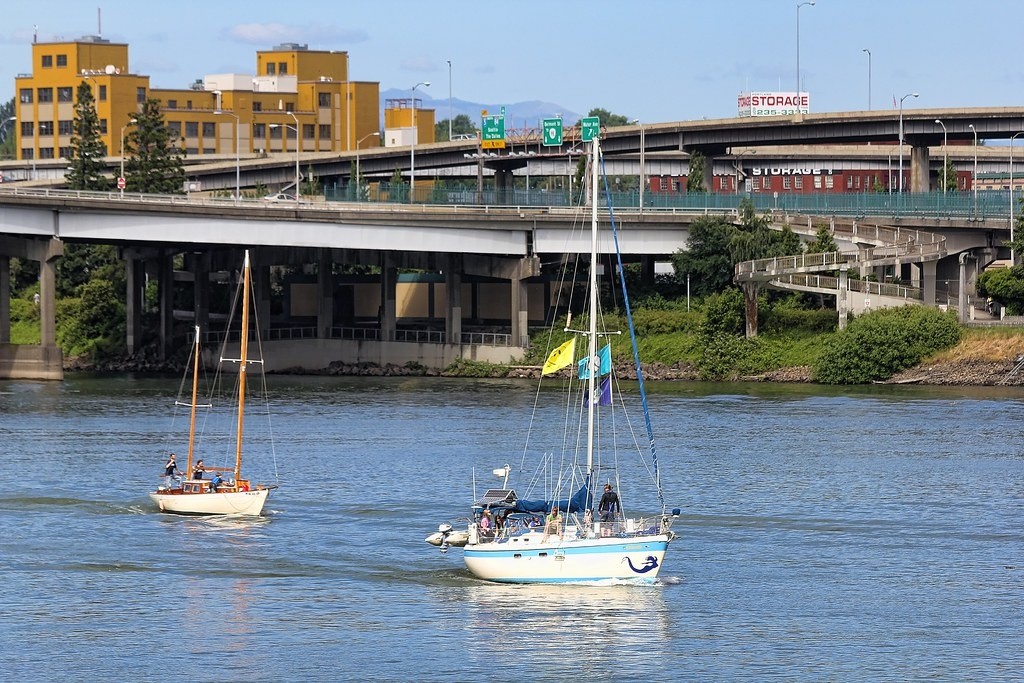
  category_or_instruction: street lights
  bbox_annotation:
[357,132,380,202]
[968,125,977,222]
[862,48,872,111]
[889,141,906,207]
[33,125,46,181]
[935,119,947,207]
[410,81,431,204]
[329,50,351,152]
[900,93,920,194]
[446,60,452,142]
[796,1,816,112]
[120,118,138,199]
[214,111,240,199]
[268,111,300,207]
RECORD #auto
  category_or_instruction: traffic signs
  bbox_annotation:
[582,116,600,142]
[543,118,562,146]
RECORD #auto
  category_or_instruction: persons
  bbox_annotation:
[33,292,40,305]
[529,516,543,526]
[166,454,183,489]
[480,510,494,542]
[598,484,620,537]
[194,460,214,479]
[987,296,994,315]
[495,515,507,539]
[207,474,226,493]
[544,506,563,540]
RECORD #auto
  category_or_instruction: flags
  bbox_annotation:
[578,343,611,379]
[542,338,575,375]
[585,375,612,408]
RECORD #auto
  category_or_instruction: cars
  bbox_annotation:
[450,133,482,145]
[258,193,295,204]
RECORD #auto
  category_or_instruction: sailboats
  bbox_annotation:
[145,250,279,515]
[424,132,681,585]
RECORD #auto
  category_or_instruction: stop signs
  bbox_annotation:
[118,177,126,189]
